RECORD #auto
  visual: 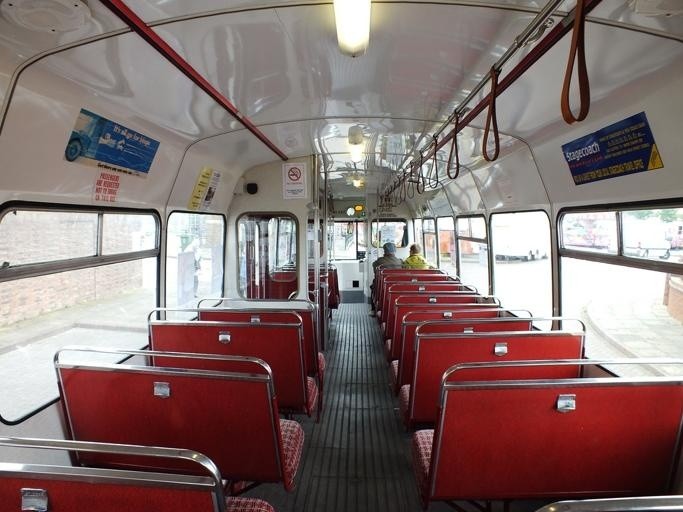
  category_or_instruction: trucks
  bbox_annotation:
[493,250,549,262]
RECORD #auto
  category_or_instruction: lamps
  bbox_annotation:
[333,0,370,58]
[352,161,361,187]
[347,123,367,145]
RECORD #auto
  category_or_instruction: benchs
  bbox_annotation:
[1,437,275,512]
[411,355,682,502]
[195,298,327,385]
[536,495,683,511]
[263,265,341,324]
[146,307,320,418]
[54,348,305,492]
[369,262,534,385]
[395,318,587,431]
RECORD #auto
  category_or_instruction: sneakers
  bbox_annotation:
[368,307,375,317]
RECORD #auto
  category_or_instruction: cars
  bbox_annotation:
[608,241,671,260]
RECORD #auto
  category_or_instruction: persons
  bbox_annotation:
[526,250,532,261]
[366,242,404,318]
[99,131,126,154]
[401,243,431,269]
[533,249,541,260]
[191,245,204,300]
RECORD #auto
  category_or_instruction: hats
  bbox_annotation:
[382,242,396,253]
